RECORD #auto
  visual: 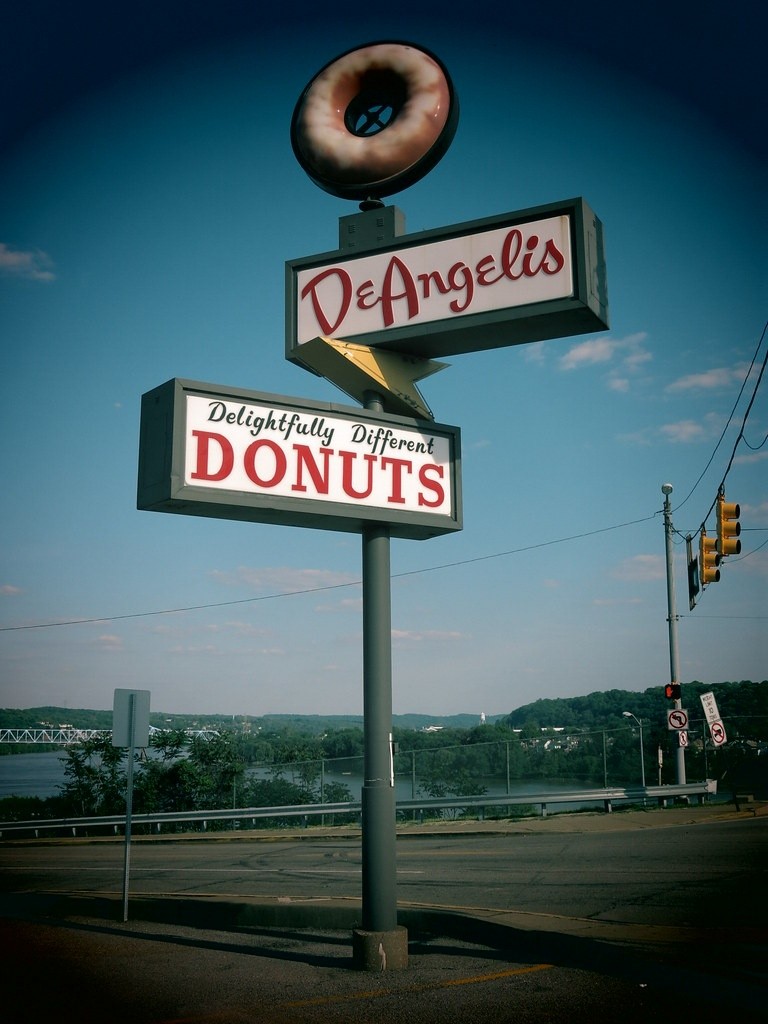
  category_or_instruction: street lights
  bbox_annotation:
[624,712,647,808]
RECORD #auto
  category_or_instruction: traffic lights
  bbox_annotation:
[716,494,741,556]
[699,528,722,585]
[664,683,682,700]
